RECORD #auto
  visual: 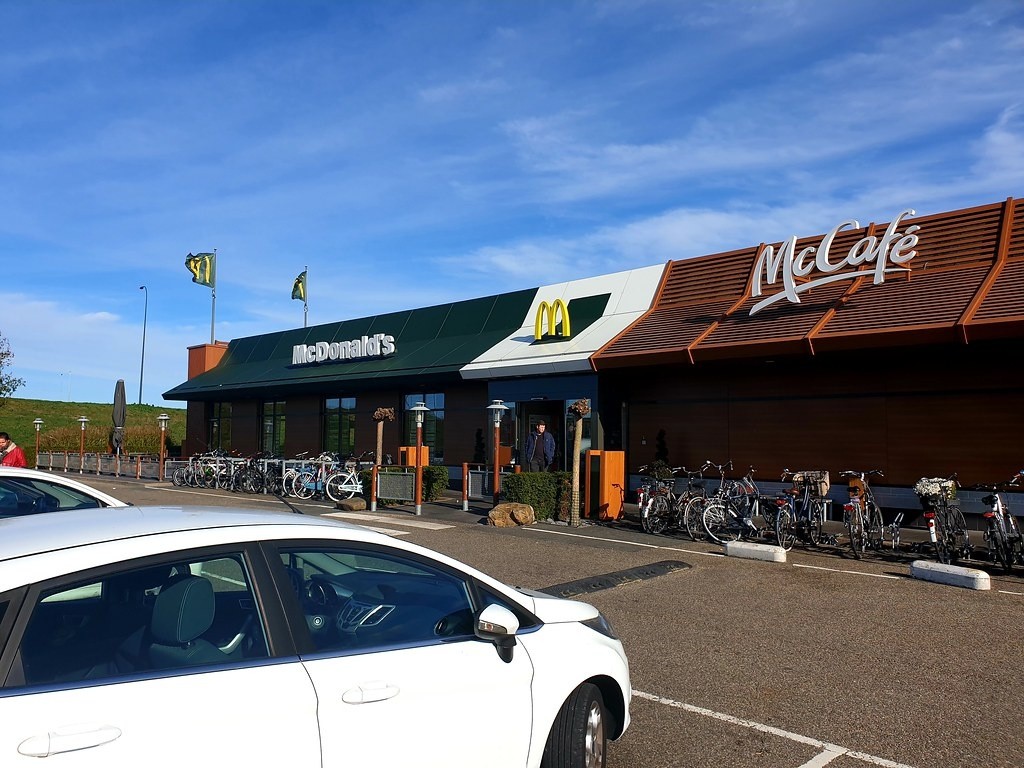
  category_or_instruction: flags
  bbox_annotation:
[185,252,216,288]
[291,271,306,301]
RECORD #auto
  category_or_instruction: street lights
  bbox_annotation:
[485,400,509,511]
[409,401,430,516]
[138,284,148,405]
[76,415,90,475]
[33,417,45,470]
[156,414,171,483]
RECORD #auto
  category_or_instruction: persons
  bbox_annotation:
[526,420,556,472]
[0,432,28,468]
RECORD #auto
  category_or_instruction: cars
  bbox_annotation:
[0,468,131,519]
[0,504,633,765]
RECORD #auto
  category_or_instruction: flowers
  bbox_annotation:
[373,407,396,421]
[568,397,592,418]
[913,477,957,500]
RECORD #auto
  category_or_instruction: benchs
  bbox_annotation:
[755,493,832,522]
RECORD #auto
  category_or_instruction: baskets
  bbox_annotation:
[918,481,955,510]
[793,470,830,497]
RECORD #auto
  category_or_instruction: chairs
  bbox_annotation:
[137,561,237,669]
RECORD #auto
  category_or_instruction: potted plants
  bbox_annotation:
[650,460,675,494]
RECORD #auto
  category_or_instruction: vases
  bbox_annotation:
[920,498,961,530]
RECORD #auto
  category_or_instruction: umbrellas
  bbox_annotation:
[110,379,128,477]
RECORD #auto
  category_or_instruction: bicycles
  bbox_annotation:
[970,469,1024,576]
[912,470,977,566]
[636,459,886,560]
[171,446,393,502]
